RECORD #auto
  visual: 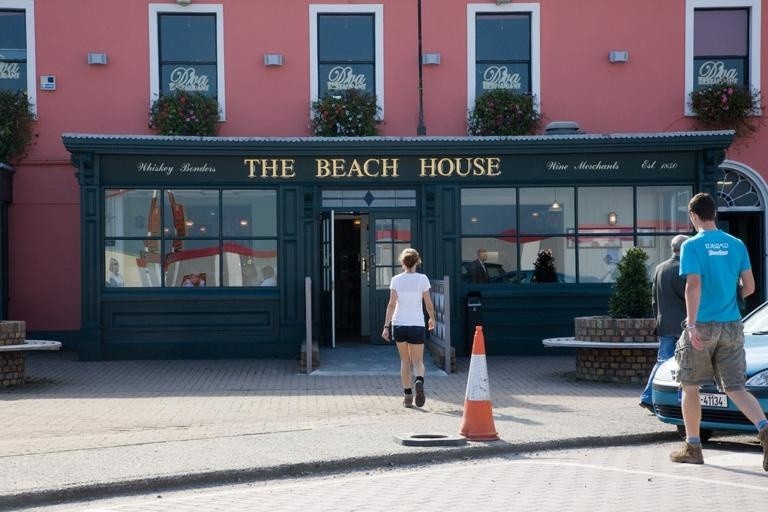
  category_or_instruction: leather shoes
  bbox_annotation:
[639,400,655,414]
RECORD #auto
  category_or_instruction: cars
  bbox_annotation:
[651,301,768,445]
[462,260,506,284]
[485,270,573,284]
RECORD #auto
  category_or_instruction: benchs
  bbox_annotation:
[540,332,663,357]
[0,336,67,355]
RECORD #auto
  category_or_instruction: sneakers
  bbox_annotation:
[756,423,767,472]
[414,380,425,407]
[402,394,412,407]
[669,439,704,465]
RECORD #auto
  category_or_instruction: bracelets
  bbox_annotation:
[382,325,390,328]
[685,323,696,330]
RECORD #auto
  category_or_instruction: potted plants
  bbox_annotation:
[572,247,660,389]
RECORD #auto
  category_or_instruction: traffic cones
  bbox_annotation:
[456,325,501,442]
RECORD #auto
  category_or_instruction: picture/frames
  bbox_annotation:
[566,226,656,249]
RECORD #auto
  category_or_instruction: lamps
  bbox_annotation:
[547,187,562,212]
[606,210,618,227]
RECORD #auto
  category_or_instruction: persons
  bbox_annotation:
[639,234,690,413]
[259,265,278,286]
[471,247,489,284]
[380,248,437,407]
[669,193,767,471]
[599,253,622,282]
[107,256,125,287]
[182,271,205,288]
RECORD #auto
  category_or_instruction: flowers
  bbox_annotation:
[1,85,42,169]
[687,77,759,138]
[146,86,222,138]
[305,89,377,138]
[465,89,543,138]
